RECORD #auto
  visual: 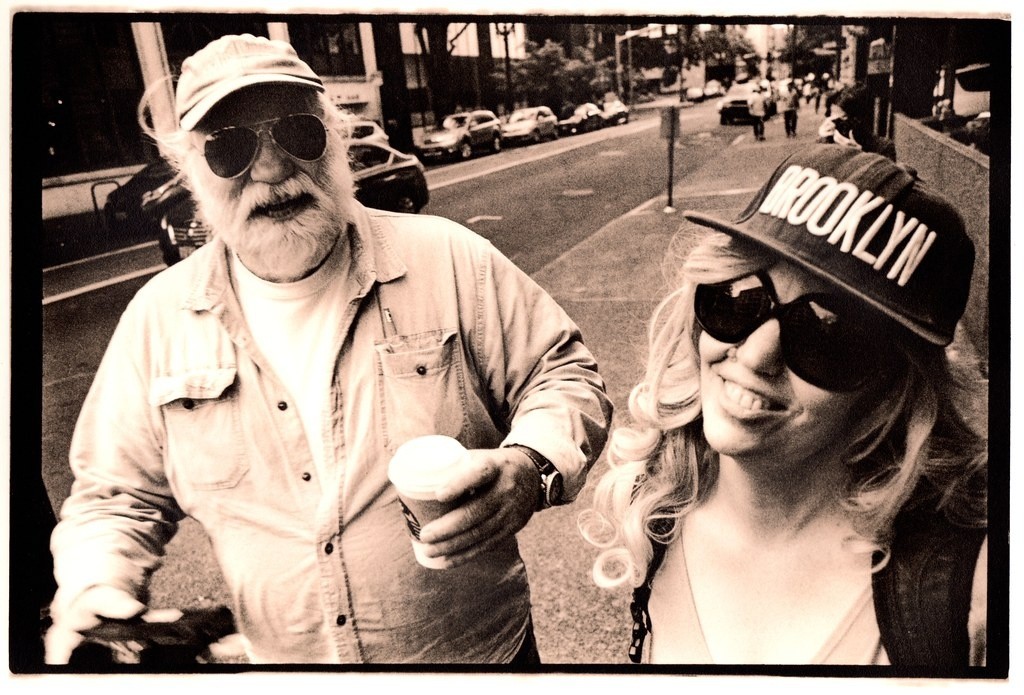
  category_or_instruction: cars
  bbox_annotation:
[687,86,703,102]
[931,63,993,121]
[338,120,390,152]
[161,141,429,271]
[703,81,722,98]
[98,161,191,235]
[598,100,631,126]
[558,103,607,135]
[499,107,560,145]
[718,81,764,122]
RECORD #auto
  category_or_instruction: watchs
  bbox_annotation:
[510,445,563,509]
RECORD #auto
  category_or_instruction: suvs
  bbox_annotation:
[414,109,503,162]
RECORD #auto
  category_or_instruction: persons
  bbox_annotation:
[43,36,611,664]
[748,73,896,165]
[578,146,988,664]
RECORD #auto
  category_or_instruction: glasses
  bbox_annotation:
[190,112,326,179]
[693,268,896,394]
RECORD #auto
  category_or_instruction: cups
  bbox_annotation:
[388,435,472,570]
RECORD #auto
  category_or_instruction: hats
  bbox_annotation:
[681,142,975,346]
[176,32,324,131]
